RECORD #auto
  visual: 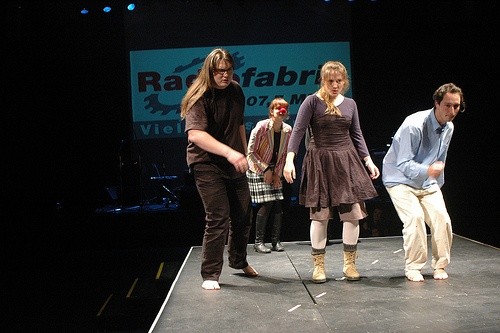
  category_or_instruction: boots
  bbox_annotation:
[254,212,271,254]
[272,213,284,252]
[342,244,359,281]
[311,248,326,284]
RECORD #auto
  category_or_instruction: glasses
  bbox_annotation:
[213,67,233,75]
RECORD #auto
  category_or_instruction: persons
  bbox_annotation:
[181,49,258,290]
[283,61,380,282]
[382,83,463,282]
[246,98,292,253]
